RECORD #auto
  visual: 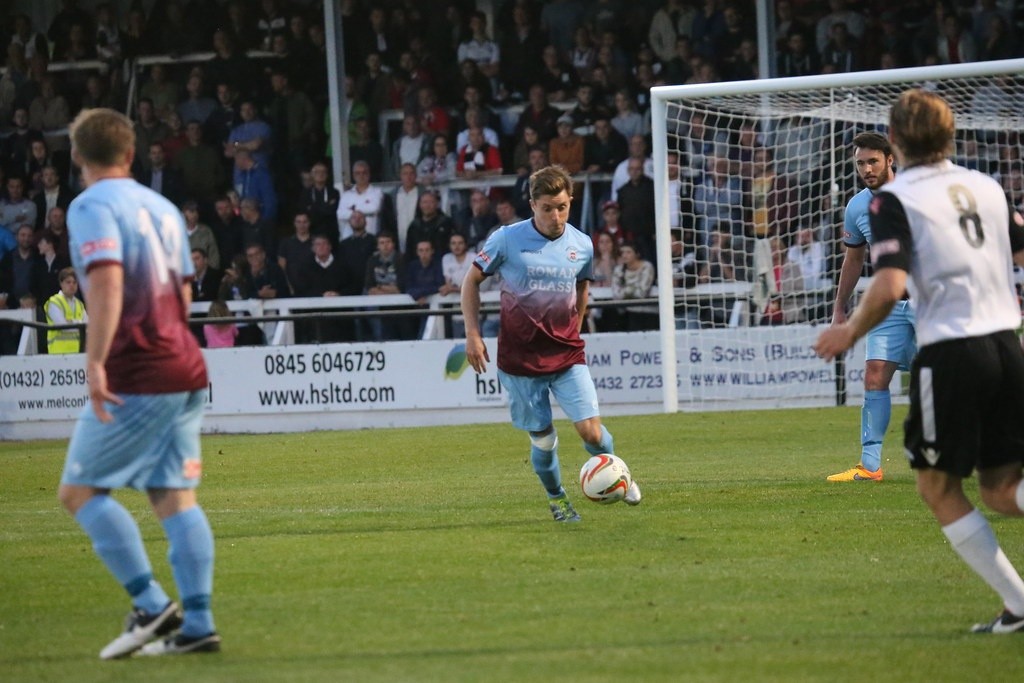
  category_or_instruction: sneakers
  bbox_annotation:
[970,606,1024,634]
[621,480,643,507]
[99,600,185,661]
[826,462,883,482]
[545,486,582,523]
[137,628,222,657]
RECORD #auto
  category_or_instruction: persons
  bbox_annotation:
[611,134,653,204]
[44,268,90,355]
[512,149,546,220]
[456,124,503,199]
[56,106,223,660]
[827,130,919,483]
[391,114,430,175]
[1,1,1024,351]
[695,154,745,259]
[548,116,585,177]
[416,133,457,218]
[200,300,240,350]
[456,10,500,98]
[336,160,385,241]
[811,89,1024,634]
[461,163,642,521]
[390,163,426,257]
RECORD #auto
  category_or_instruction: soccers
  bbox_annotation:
[580,452,633,505]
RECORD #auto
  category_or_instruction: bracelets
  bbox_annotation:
[235,140,239,148]
